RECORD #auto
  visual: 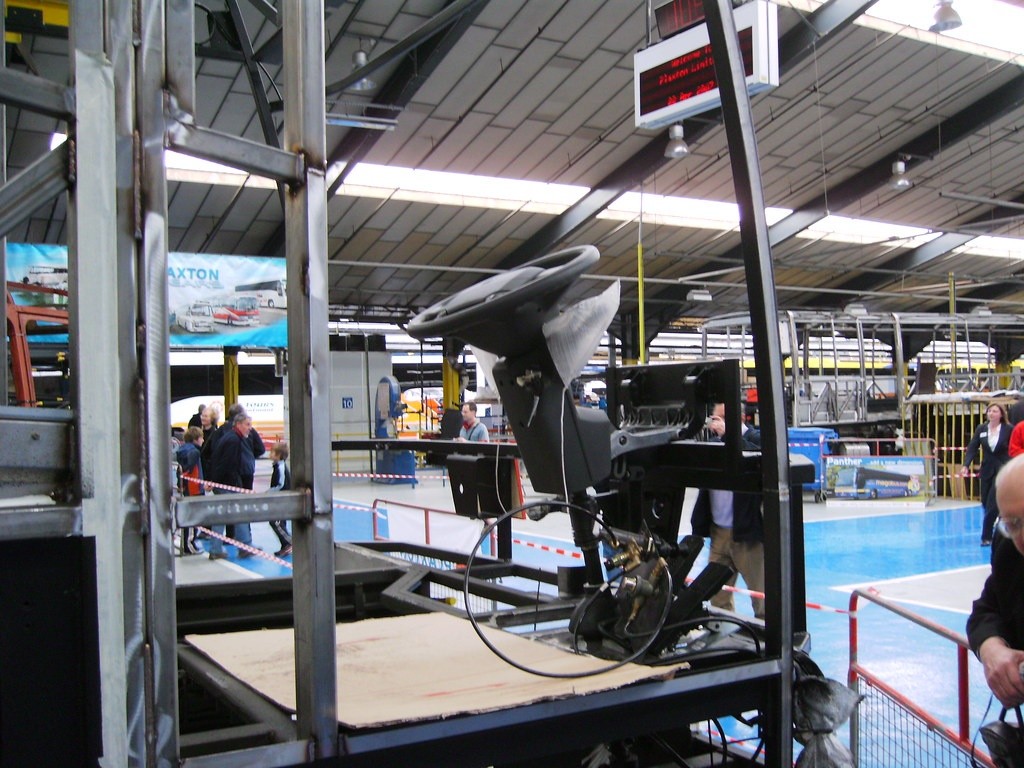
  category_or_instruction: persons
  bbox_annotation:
[691,405,764,621]
[958,397,1024,716]
[174,404,293,561]
[866,424,906,454]
[456,401,490,444]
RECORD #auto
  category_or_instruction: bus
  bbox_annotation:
[235,280,287,309]
[21,265,67,284]
[832,467,917,501]
[174,301,214,334]
[212,295,259,327]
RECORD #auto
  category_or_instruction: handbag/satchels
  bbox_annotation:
[980,699,1024,768]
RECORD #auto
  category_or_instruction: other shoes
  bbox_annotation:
[186,550,205,555]
[980,539,991,546]
[238,547,264,559]
[209,552,229,560]
[275,543,293,557]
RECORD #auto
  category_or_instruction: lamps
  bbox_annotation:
[664,123,691,159]
[347,38,376,92]
[928,0,962,32]
[888,157,911,191]
